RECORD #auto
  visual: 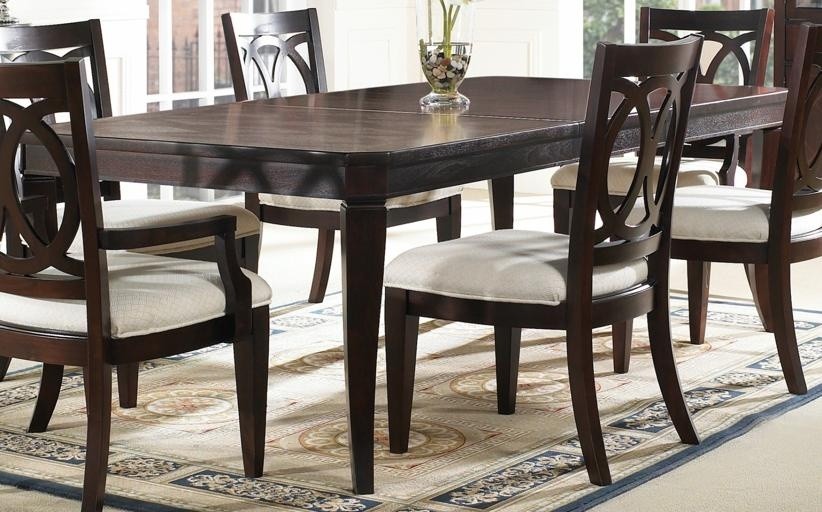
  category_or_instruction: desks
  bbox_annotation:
[16,75,788,494]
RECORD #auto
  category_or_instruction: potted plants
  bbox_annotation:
[418,0,473,109]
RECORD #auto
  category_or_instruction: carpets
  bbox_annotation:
[2,275,822,511]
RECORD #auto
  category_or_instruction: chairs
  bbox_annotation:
[551,6,775,235]
[221,7,462,304]
[382,33,705,486]
[612,23,822,395]
[0,57,273,511]
[2,19,262,409]
